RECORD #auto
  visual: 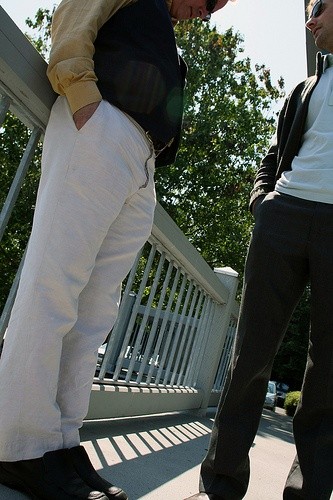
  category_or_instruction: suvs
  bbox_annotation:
[276,381,293,405]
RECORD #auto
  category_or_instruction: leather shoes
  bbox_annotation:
[0,448,109,500]
[69,446,129,500]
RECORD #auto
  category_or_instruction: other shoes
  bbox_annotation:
[184,491,224,500]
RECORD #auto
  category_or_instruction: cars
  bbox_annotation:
[96,340,161,373]
[264,380,278,410]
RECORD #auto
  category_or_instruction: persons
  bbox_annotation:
[199,0,333,500]
[0,0,229,500]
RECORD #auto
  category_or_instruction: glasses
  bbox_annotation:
[206,0,217,18]
[308,0,323,19]
